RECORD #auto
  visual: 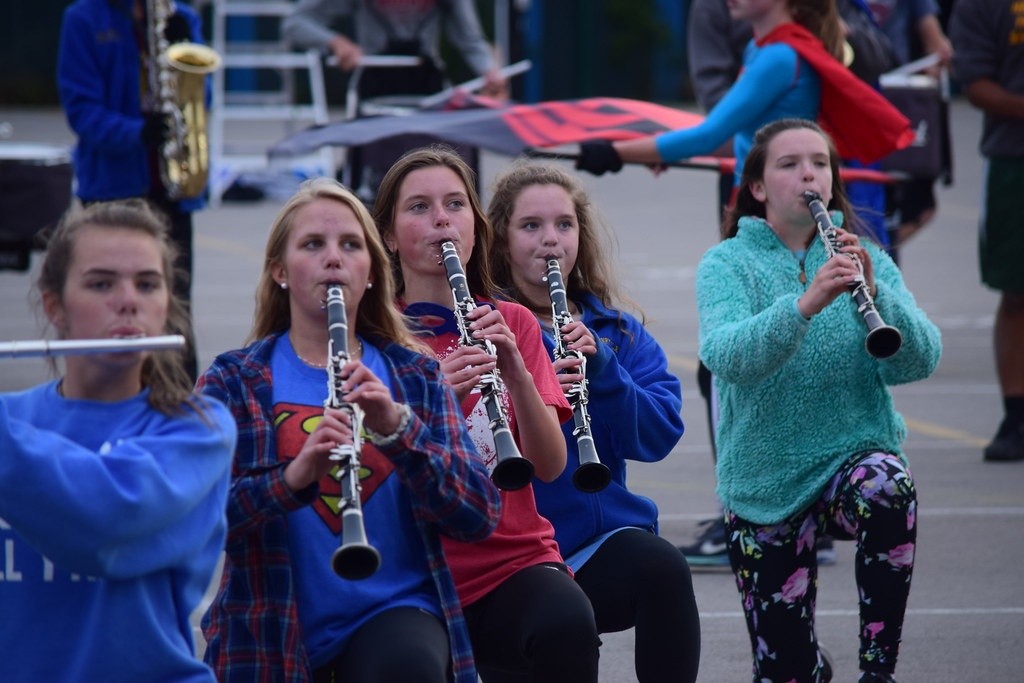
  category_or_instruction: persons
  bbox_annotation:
[372,146,603,683]
[697,120,944,683]
[0,198,238,683]
[61,0,1024,572]
[194,181,500,683]
[488,168,701,683]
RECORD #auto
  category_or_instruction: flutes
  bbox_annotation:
[435,240,537,491]
[321,282,382,582]
[805,189,901,358]
[542,254,614,493]
[0,334,185,358]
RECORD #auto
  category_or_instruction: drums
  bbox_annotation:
[358,96,472,199]
[869,75,948,186]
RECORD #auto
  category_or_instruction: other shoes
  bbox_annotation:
[985,420,1024,461]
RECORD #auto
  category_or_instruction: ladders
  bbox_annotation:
[210,0,337,216]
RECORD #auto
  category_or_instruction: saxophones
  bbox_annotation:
[147,0,223,198]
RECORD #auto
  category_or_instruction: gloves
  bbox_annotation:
[575,139,623,176]
[139,109,171,153]
[163,7,193,44]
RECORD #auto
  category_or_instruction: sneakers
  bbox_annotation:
[680,517,731,566]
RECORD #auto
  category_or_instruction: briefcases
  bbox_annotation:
[879,54,955,185]
